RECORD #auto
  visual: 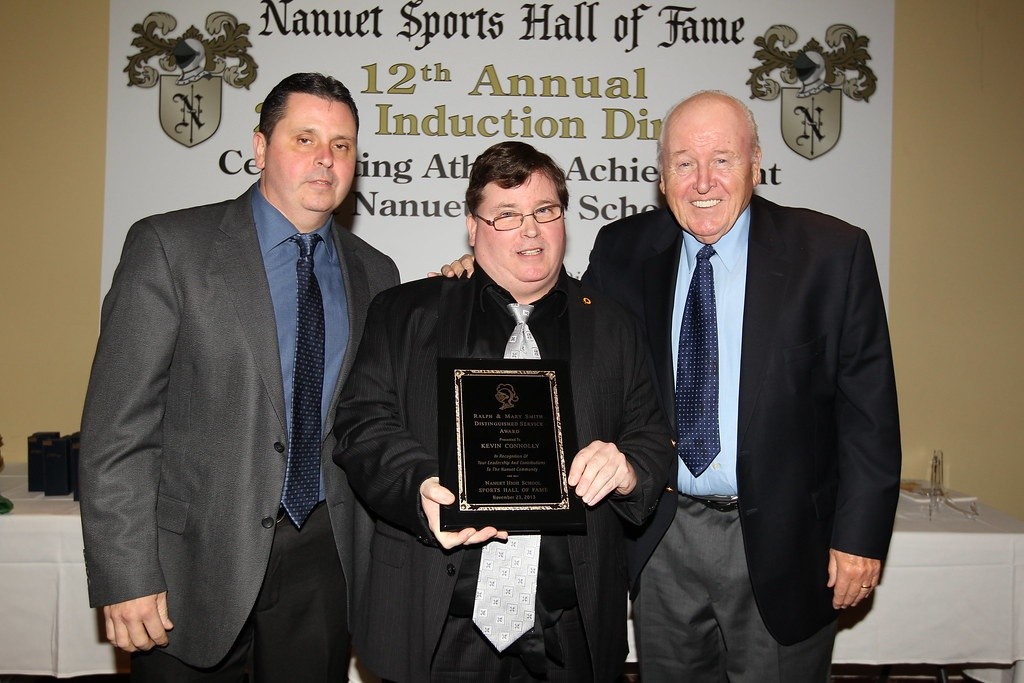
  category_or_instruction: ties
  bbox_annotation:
[473,302,544,653]
[280,233,325,528]
[676,244,722,478]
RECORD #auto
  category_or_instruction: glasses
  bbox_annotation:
[474,203,564,232]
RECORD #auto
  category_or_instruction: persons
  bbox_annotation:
[78,71,402,683]
[427,90,901,683]
[332,141,674,682]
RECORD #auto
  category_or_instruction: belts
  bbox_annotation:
[677,493,738,512]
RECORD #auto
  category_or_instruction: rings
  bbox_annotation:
[861,586,871,588]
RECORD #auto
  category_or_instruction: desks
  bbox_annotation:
[0,462,1024,683]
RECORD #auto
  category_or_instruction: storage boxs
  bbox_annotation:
[28,432,80,496]
